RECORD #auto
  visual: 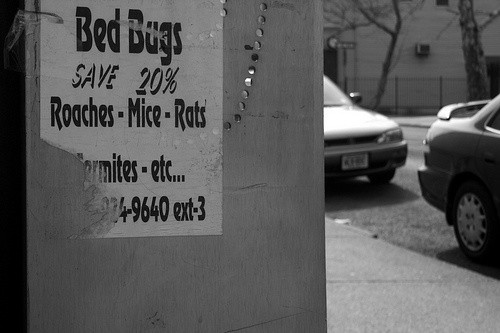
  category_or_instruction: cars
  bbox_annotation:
[415,90,500,260]
[321,74,409,190]
